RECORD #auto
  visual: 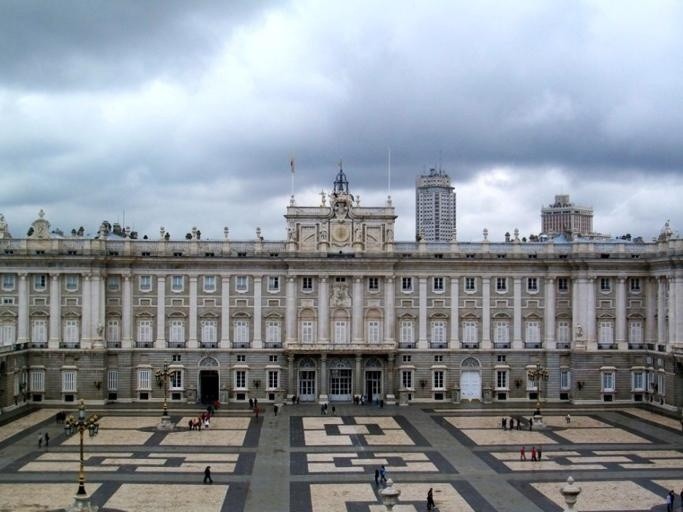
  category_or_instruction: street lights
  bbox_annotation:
[62,398,101,498]
[153,361,176,417]
[528,360,549,410]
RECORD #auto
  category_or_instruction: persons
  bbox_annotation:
[188,400,221,431]
[375,469,379,487]
[37,432,42,449]
[203,466,213,483]
[502,417,532,432]
[320,403,337,416]
[519,446,542,461]
[427,487,435,512]
[666,489,683,512]
[44,432,50,447]
[379,465,387,483]
[352,394,365,408]
[65,415,98,441]
[249,397,261,419]
